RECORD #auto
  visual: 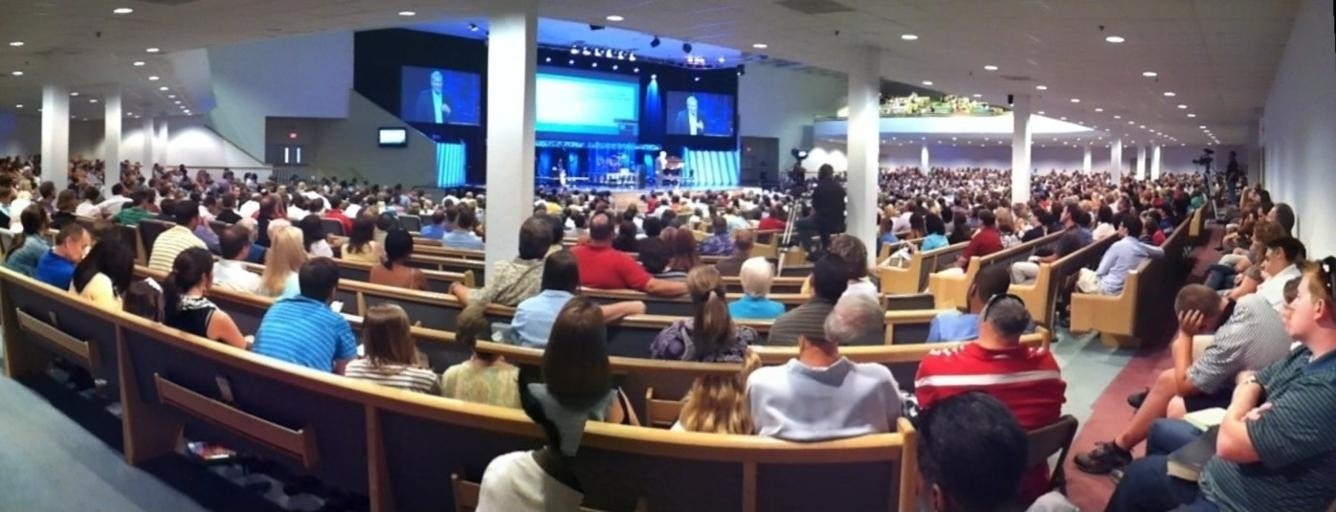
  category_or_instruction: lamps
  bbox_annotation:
[682,43,692,53]
[471,24,479,32]
[569,44,637,63]
[651,36,661,47]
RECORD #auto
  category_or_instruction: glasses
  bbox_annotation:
[983,291,1026,326]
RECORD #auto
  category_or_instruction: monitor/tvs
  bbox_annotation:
[535,63,640,141]
[664,89,737,151]
[400,65,483,130]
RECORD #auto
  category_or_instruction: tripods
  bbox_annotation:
[777,198,810,277]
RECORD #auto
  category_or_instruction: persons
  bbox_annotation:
[2,148,1335,512]
[674,95,707,136]
[417,70,455,125]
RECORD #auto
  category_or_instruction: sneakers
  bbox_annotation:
[1126,386,1148,411]
[1074,438,1134,475]
